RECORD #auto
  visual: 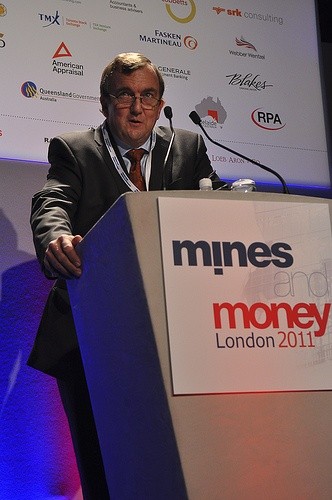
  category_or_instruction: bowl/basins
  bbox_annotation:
[231,178,256,191]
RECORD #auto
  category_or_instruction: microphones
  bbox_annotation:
[161,105,176,190]
[189,110,291,194]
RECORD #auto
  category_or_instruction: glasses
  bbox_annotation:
[108,92,161,110]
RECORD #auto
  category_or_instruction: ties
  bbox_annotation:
[127,148,147,191]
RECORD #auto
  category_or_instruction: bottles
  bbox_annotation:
[198,178,213,191]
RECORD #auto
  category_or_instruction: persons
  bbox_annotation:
[26,53,222,500]
